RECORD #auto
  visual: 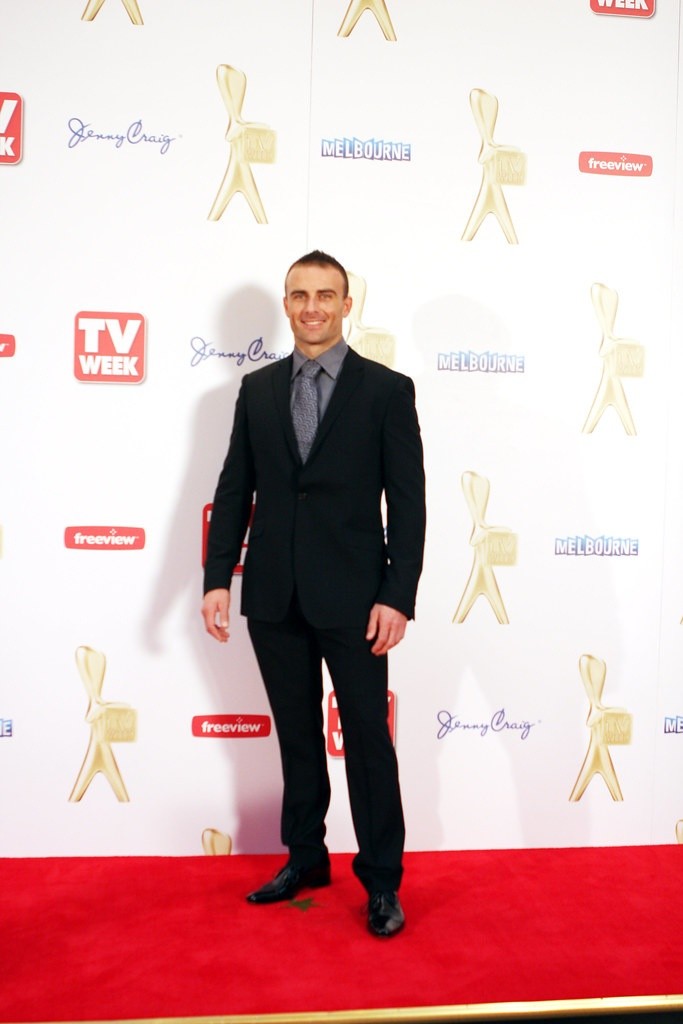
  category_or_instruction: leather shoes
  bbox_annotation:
[368,891,405,937]
[246,863,330,904]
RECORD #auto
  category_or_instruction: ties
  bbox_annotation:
[292,360,321,464]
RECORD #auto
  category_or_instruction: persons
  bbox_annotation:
[201,250,427,936]
